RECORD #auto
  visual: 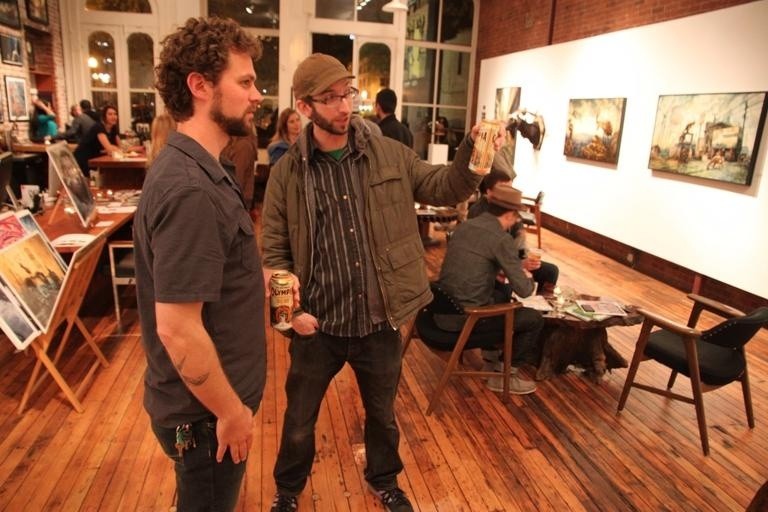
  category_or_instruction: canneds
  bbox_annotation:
[469,119,501,175]
[268,271,295,332]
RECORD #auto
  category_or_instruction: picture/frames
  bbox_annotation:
[0,32,24,66]
[5,76,31,121]
[25,0,48,26]
[0,0,21,29]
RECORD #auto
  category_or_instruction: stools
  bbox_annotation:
[109,240,135,321]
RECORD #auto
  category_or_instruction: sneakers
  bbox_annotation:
[480,362,537,394]
[269,492,298,512]
[366,483,414,512]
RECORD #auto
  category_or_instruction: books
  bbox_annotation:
[507,280,629,324]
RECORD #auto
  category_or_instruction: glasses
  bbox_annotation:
[306,86,359,105]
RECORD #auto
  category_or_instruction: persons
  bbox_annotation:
[74,105,145,179]
[29,98,58,138]
[375,88,413,150]
[145,114,177,169]
[466,169,558,295]
[260,52,506,512]
[80,99,101,122]
[57,103,97,145]
[436,185,544,394]
[132,95,152,144]
[134,18,301,512]
[253,98,273,148]
[267,108,301,167]
[219,122,258,212]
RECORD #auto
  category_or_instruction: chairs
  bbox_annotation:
[618,294,768,456]
[520,191,545,249]
[415,281,521,415]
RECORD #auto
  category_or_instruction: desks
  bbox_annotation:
[88,154,150,187]
[512,282,642,385]
[13,153,48,198]
[32,188,141,254]
[414,202,457,248]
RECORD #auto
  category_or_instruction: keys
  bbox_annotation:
[175,424,183,457]
[183,425,191,451]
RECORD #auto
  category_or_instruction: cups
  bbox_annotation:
[525,248,543,271]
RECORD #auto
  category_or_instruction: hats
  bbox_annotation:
[293,53,355,98]
[487,184,531,211]
